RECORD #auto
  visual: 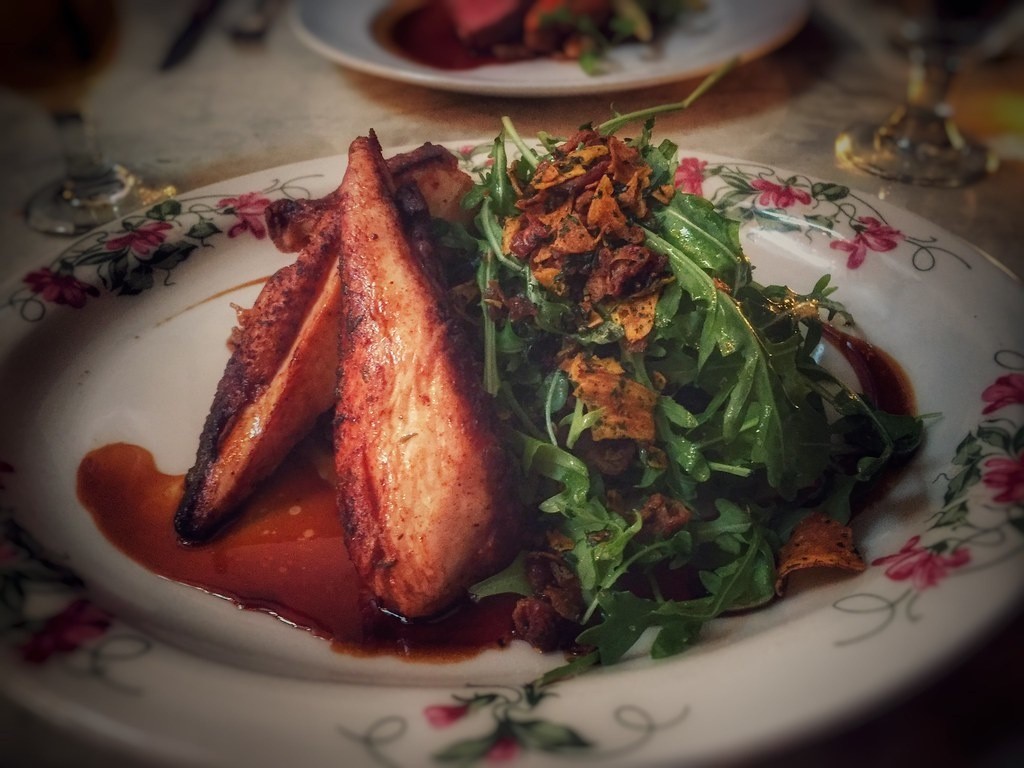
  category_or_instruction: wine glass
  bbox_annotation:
[0,0,179,238]
[818,0,1024,191]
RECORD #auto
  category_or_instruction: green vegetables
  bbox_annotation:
[419,47,947,768]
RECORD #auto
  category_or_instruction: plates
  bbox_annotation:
[288,0,810,97]
[0,136,1024,768]
